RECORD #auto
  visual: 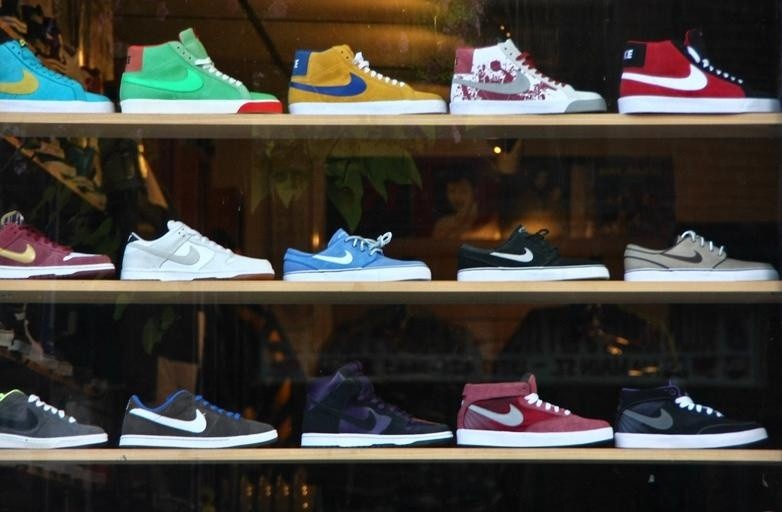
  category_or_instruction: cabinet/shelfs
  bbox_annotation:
[0,111,782,463]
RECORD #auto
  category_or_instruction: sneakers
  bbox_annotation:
[457,372,613,450]
[457,226,610,279]
[118,28,282,113]
[450,39,607,112]
[625,232,777,281]
[0,38,113,112]
[119,391,278,448]
[121,221,275,281]
[288,44,447,113]
[0,210,116,279]
[617,32,779,113]
[282,228,431,281]
[616,380,768,448]
[0,390,107,448]
[297,360,454,446]
